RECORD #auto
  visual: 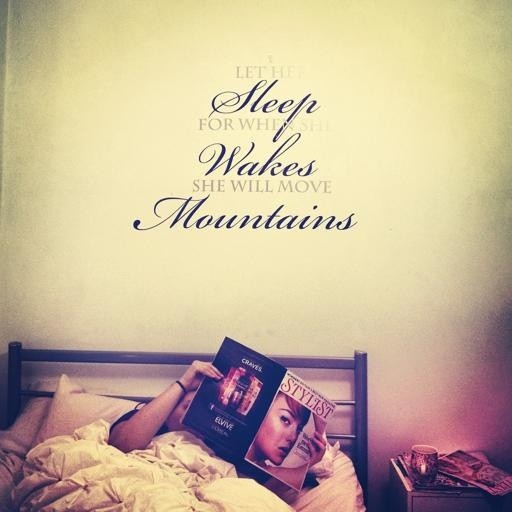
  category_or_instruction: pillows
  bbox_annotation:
[28,370,155,452]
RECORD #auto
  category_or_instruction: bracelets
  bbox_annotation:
[175,380,188,395]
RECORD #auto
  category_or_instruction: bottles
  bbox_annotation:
[228,371,252,410]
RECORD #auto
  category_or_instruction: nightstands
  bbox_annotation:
[388,448,504,512]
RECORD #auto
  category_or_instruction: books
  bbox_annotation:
[179,335,337,492]
[395,445,511,496]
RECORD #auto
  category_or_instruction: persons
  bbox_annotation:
[243,387,326,491]
[106,353,329,503]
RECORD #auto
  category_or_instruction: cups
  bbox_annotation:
[409,444,439,483]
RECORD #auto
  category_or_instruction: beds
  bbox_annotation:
[0,340,370,512]
